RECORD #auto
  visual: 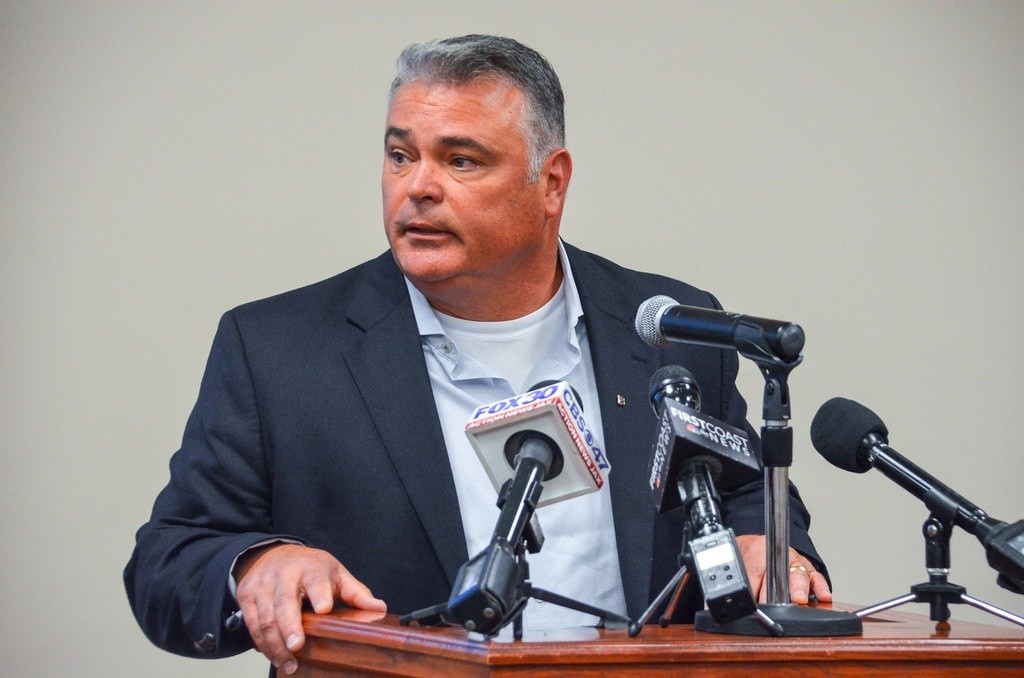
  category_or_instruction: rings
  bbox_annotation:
[789,565,807,575]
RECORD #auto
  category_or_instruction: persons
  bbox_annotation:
[120,34,832,678]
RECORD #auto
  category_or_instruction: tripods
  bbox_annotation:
[852,511,1024,626]
[399,536,633,640]
[629,520,784,639]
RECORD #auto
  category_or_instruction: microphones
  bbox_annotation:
[811,396,1024,597]
[646,364,764,625]
[449,379,611,634]
[634,295,806,362]
